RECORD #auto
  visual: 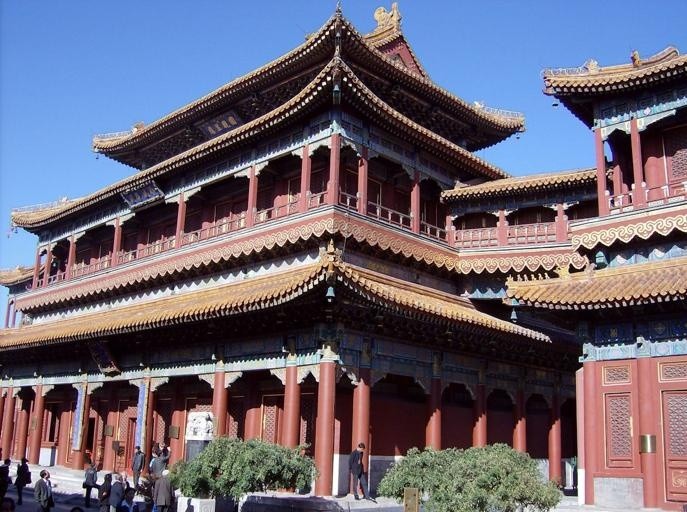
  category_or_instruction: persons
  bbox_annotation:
[0,457,55,512]
[348,443,371,500]
[70,442,174,512]
